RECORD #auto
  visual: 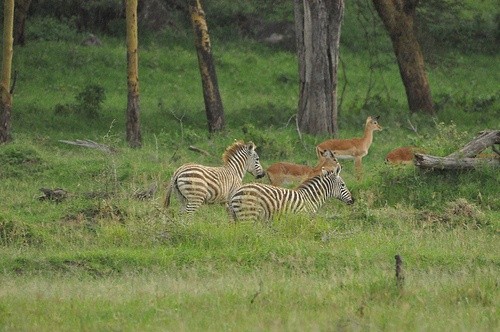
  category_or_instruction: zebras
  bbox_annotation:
[230,162,355,224]
[163,139,265,215]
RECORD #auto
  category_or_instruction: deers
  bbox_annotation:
[266,116,424,187]
